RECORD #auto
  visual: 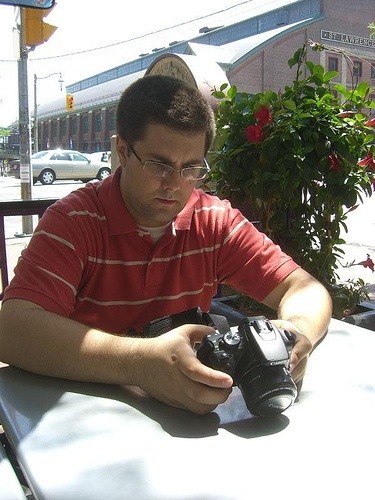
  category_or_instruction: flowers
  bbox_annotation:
[200,27,375,315]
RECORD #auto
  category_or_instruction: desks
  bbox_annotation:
[0,318,375,500]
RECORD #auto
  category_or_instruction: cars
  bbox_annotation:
[8,149,111,185]
[91,152,112,163]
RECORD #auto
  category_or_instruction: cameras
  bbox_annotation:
[193,315,298,416]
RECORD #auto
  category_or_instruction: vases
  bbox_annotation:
[209,282,375,332]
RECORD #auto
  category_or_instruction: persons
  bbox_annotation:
[0,75,334,417]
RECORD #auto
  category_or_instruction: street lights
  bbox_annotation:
[34,72,65,154]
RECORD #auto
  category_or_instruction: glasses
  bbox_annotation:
[122,137,211,180]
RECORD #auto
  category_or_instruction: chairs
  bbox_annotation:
[0,197,60,301]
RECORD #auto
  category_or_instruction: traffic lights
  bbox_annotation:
[65,94,73,112]
[19,2,57,49]
